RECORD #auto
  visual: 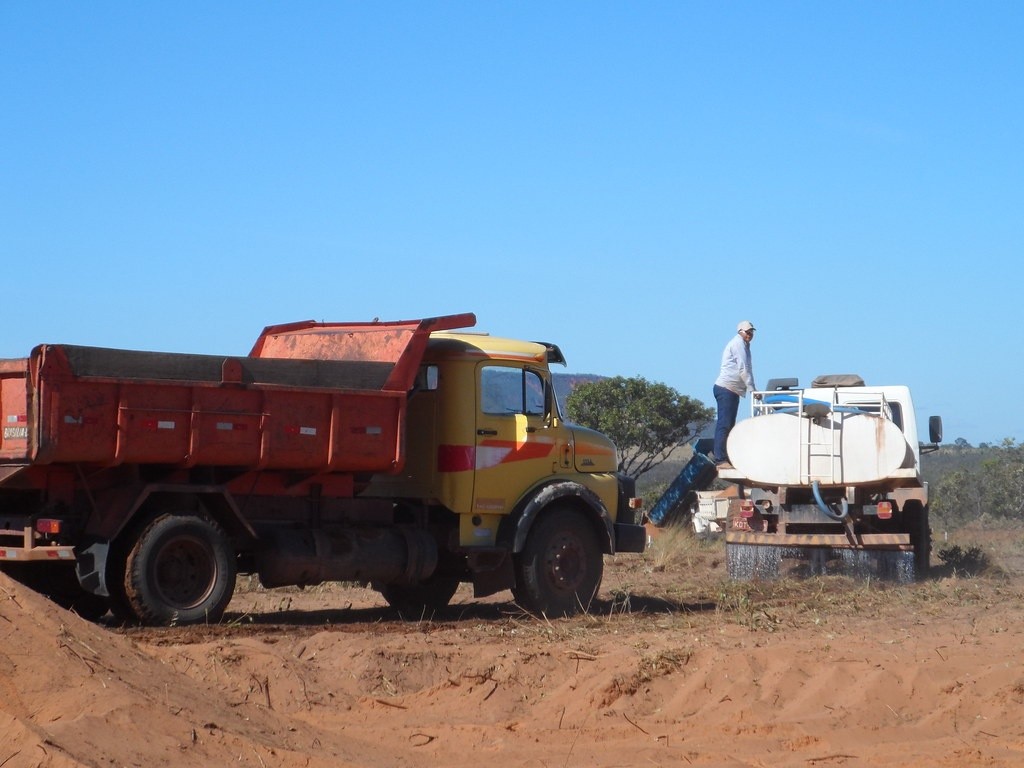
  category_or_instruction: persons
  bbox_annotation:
[713,321,762,469]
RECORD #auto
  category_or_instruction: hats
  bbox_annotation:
[737,321,756,332]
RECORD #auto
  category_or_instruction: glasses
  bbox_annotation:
[740,329,755,334]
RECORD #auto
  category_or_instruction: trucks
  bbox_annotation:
[0,312,647,632]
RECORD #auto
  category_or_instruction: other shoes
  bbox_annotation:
[715,461,732,469]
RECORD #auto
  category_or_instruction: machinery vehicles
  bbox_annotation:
[712,376,943,584]
